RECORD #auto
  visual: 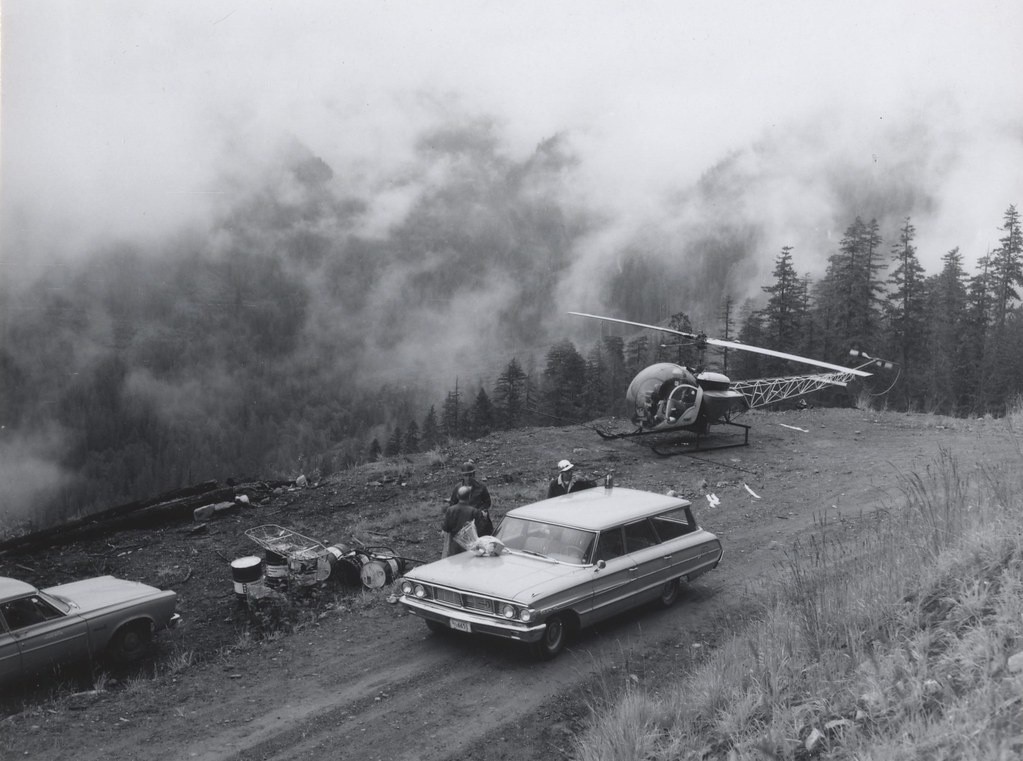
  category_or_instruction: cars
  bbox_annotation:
[393,472,723,670]
[0,574,184,686]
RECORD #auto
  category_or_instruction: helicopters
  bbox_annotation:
[564,309,902,457]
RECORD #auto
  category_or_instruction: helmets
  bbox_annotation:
[461,462,475,474]
[558,460,574,472]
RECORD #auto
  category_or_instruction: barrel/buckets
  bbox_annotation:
[230,544,405,606]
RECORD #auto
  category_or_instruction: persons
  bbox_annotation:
[547,460,596,498]
[448,462,491,513]
[442,485,489,558]
